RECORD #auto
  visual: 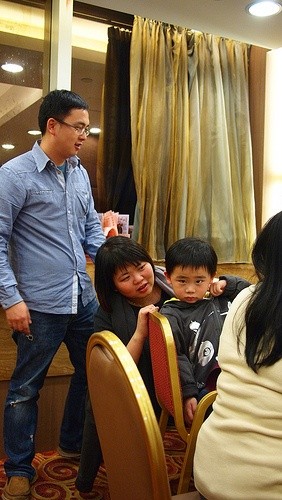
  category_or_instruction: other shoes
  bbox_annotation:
[57,445,82,457]
[1,475,31,500]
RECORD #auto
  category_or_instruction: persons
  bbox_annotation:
[159,238,252,425]
[193,212,282,500]
[93,236,186,427]
[0,89,107,500]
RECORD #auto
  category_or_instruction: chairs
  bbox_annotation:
[146,311,220,495]
[87,331,202,500]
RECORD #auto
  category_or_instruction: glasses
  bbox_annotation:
[52,116,91,137]
